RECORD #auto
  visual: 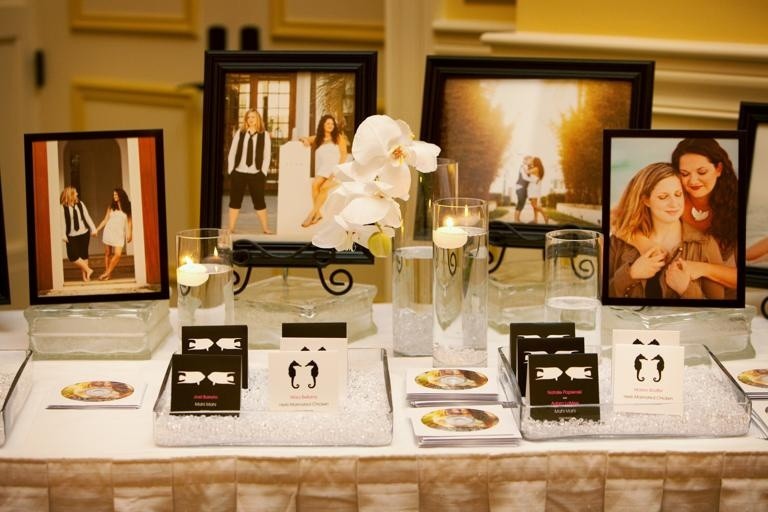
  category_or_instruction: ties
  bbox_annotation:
[246,131,257,167]
[71,204,79,231]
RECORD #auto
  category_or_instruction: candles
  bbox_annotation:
[178,264,208,288]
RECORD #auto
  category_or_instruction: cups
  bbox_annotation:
[388,157,459,358]
[545,227,604,366]
[431,197,488,369]
[175,228,234,354]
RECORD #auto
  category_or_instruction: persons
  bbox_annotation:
[60,187,97,282]
[515,156,548,224]
[92,188,132,282]
[227,108,272,235]
[608,138,737,300]
[299,115,346,228]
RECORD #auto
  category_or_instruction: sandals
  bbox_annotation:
[302,216,322,227]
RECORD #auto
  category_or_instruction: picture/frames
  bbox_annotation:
[20,127,171,307]
[597,128,746,309]
[736,100,767,287]
[412,51,654,252]
[200,47,378,268]
